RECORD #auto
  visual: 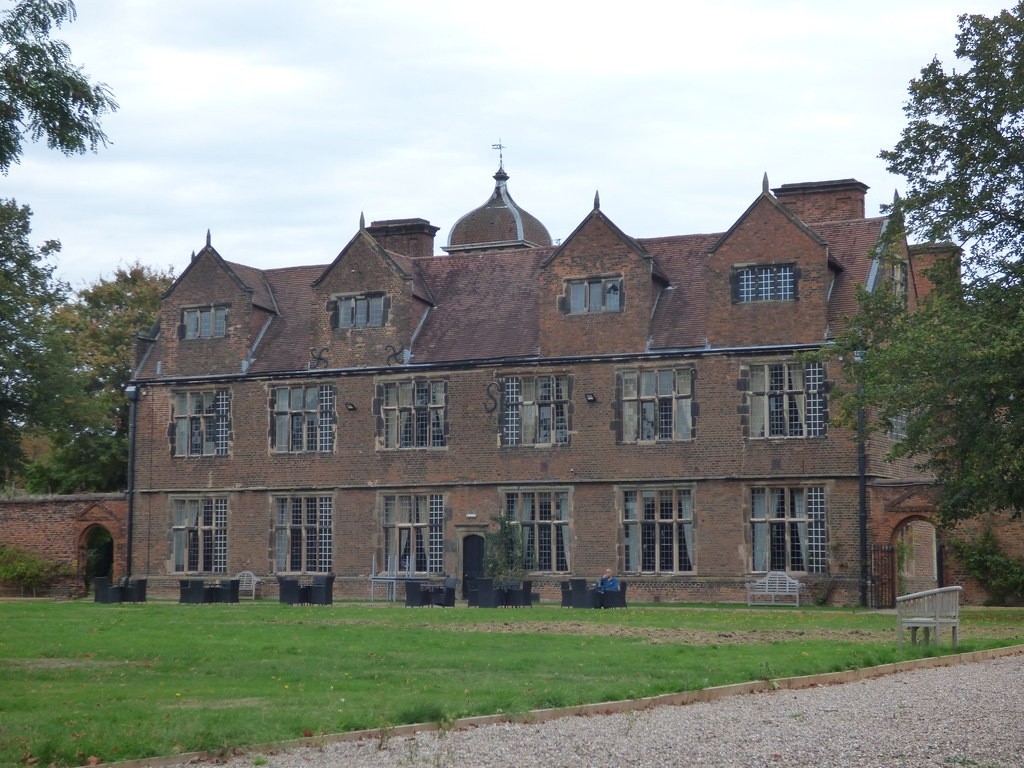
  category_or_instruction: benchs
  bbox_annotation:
[743,571,806,608]
[215,572,265,601]
[896,584,963,646]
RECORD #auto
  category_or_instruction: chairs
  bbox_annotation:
[405,577,456,608]
[466,577,533,609]
[276,573,335,606]
[177,572,240,605]
[560,579,628,610]
[94,576,147,604]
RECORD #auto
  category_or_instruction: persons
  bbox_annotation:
[595,567,622,609]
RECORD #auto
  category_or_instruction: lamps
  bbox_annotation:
[585,394,596,402]
[345,403,357,411]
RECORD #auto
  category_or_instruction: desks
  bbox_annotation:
[107,583,134,603]
[298,583,324,606]
[371,580,430,603]
[586,586,605,609]
[203,584,225,604]
[420,585,446,608]
[372,555,430,579]
[493,585,520,608]
